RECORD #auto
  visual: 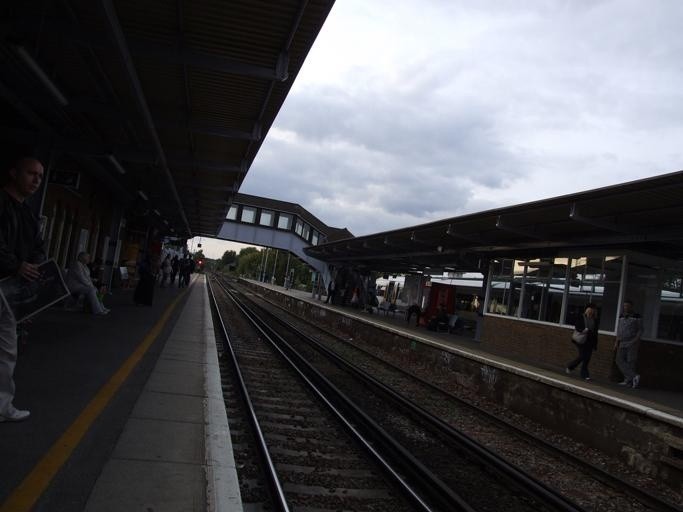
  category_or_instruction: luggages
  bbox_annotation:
[134,276,154,306]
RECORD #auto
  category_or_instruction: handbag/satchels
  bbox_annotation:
[571,330,587,344]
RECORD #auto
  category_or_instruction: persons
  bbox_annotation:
[323,278,360,307]
[613,300,643,389]
[66,252,111,316]
[565,304,598,380]
[407,303,444,331]
[271,275,277,286]
[0,155,47,422]
[134,253,196,307]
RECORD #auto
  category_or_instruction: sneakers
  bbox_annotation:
[0,410,31,422]
[100,308,110,316]
[618,374,641,388]
